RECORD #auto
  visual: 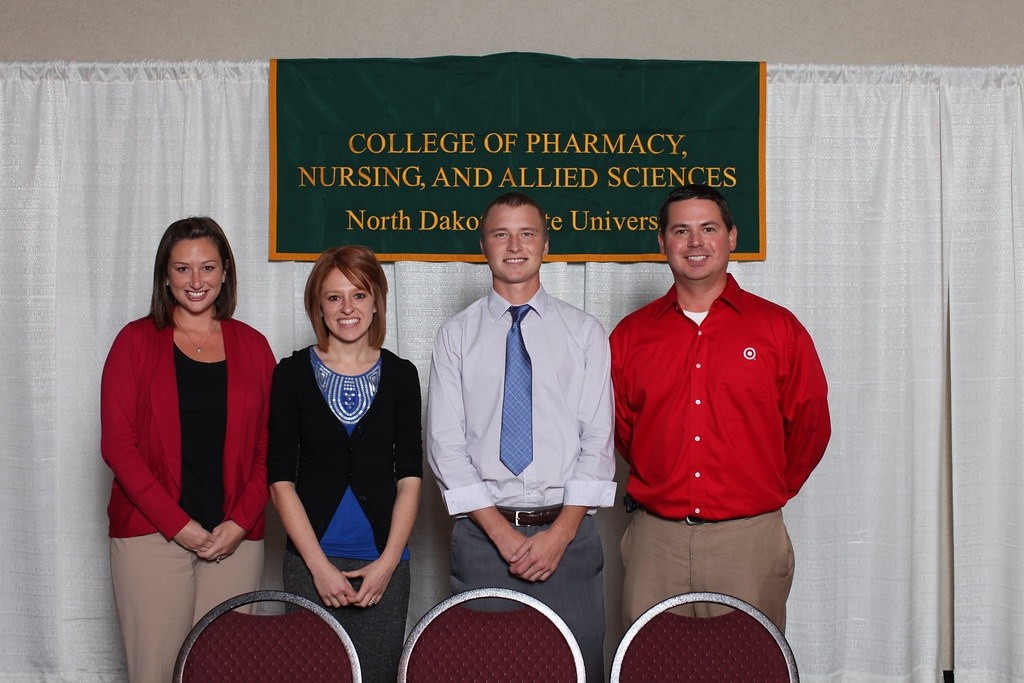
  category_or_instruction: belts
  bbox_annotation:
[498,507,563,527]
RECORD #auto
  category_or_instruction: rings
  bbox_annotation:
[216,557,220,562]
[370,599,376,603]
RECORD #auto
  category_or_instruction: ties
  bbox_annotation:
[500,304,533,478]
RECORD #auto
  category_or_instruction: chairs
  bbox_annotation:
[397,588,587,683]
[172,589,362,683]
[608,592,799,683]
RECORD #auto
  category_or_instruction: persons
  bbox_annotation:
[425,190,617,683]
[608,183,831,637]
[265,245,423,683]
[99,216,278,683]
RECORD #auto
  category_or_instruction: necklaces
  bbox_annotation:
[172,308,219,353]
